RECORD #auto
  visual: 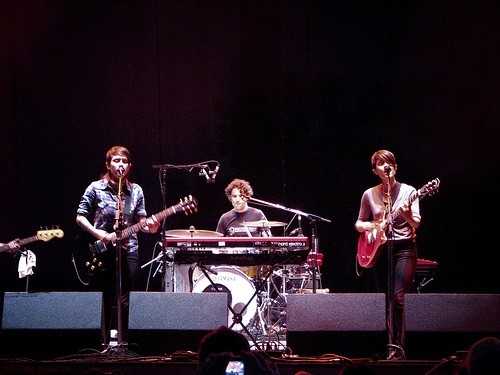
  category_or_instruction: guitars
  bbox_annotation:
[0,225,64,252]
[72,194,198,285]
[357,177,440,269]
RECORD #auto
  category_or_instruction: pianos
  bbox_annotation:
[163,237,310,263]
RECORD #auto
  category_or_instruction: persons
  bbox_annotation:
[0,240,20,253]
[76,146,160,342]
[216,179,274,237]
[356,149,421,360]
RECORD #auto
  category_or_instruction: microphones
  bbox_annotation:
[240,190,244,200]
[210,164,220,183]
[117,167,125,174]
[385,165,391,173]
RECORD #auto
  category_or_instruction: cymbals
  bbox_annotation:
[160,229,223,238]
[239,220,287,227]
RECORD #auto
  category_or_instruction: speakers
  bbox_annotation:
[402,293,500,361]
[127,291,228,357]
[2,292,106,357]
[286,293,389,359]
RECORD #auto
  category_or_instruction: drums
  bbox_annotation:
[281,266,320,291]
[191,265,257,330]
